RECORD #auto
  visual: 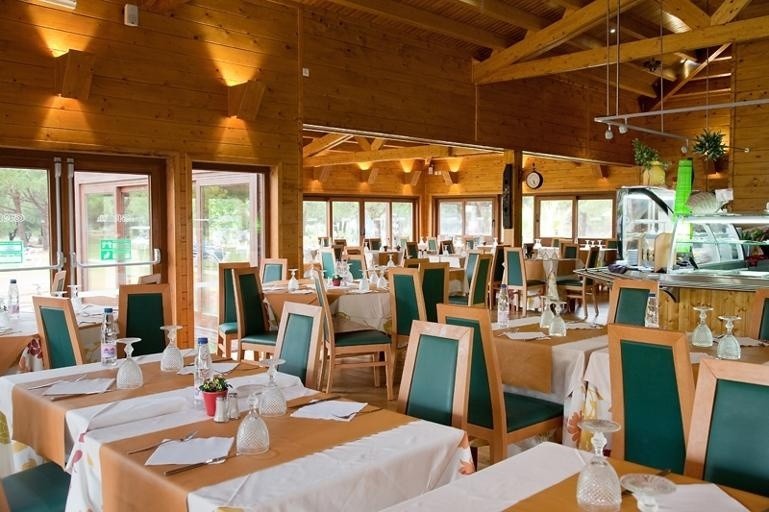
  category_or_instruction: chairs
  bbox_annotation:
[749,289,769,345]
[138,273,162,284]
[436,303,564,465]
[607,323,694,475]
[33,297,85,370]
[397,319,473,431]
[117,284,173,358]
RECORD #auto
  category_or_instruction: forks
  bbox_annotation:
[330,406,384,419]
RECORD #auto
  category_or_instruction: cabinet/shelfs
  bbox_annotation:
[664,216,769,286]
[616,185,674,266]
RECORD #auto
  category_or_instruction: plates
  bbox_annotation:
[627,249,638,266]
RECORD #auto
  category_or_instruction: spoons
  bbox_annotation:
[127,429,199,454]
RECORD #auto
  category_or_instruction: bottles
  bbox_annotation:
[213,396,229,423]
[498,285,509,328]
[645,292,660,329]
[101,308,118,365]
[193,337,214,410]
[227,393,241,419]
[8,279,19,320]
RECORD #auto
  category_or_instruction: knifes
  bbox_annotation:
[288,395,341,409]
[164,453,238,475]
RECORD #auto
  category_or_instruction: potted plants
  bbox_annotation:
[632,138,672,185]
[691,127,726,174]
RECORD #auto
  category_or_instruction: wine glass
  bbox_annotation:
[578,418,622,510]
[548,299,568,337]
[258,358,287,418]
[319,237,498,294]
[620,473,676,511]
[235,384,270,455]
[716,315,743,361]
[533,238,603,260]
[692,306,714,347]
[287,269,299,292]
[67,285,81,311]
[51,291,68,297]
[115,337,144,390]
[539,295,556,329]
[160,325,184,372]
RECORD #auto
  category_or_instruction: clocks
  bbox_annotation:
[526,171,543,189]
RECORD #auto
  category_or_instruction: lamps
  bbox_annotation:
[52,49,96,100]
[225,80,266,120]
[605,122,628,140]
[312,167,460,184]
